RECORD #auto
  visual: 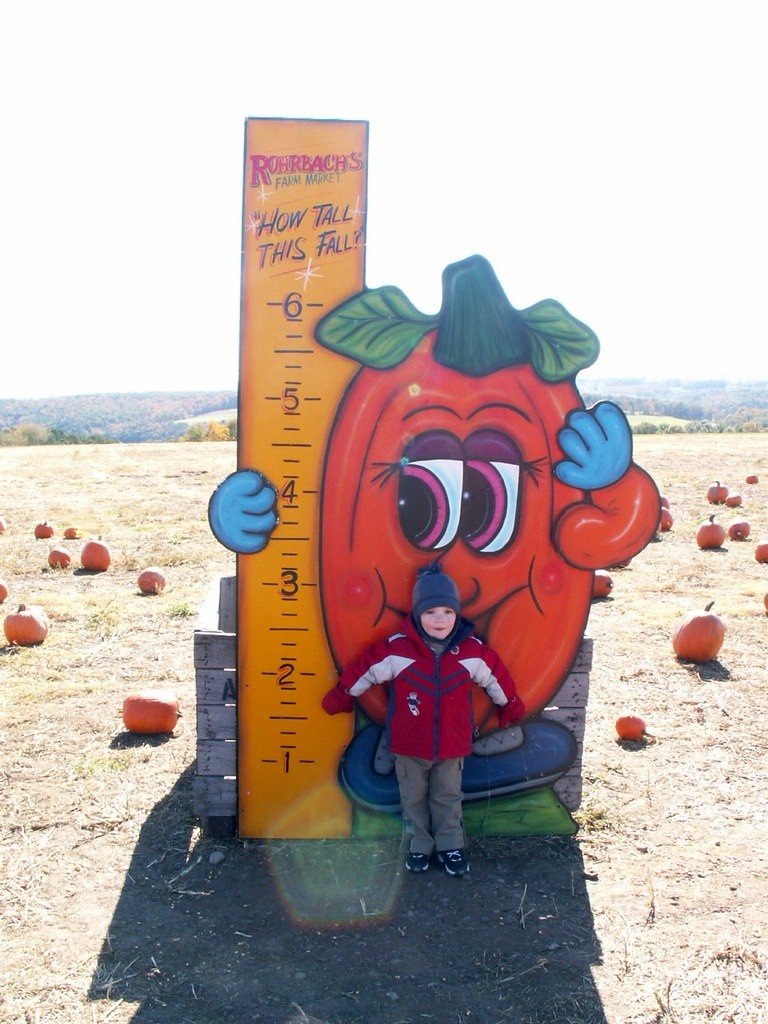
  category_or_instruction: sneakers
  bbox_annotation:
[437,850,470,876]
[405,852,429,872]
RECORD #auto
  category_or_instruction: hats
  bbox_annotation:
[412,572,460,645]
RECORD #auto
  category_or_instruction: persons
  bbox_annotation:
[319,561,527,881]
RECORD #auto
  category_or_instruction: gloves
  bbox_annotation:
[499,696,524,730]
[321,683,354,716]
